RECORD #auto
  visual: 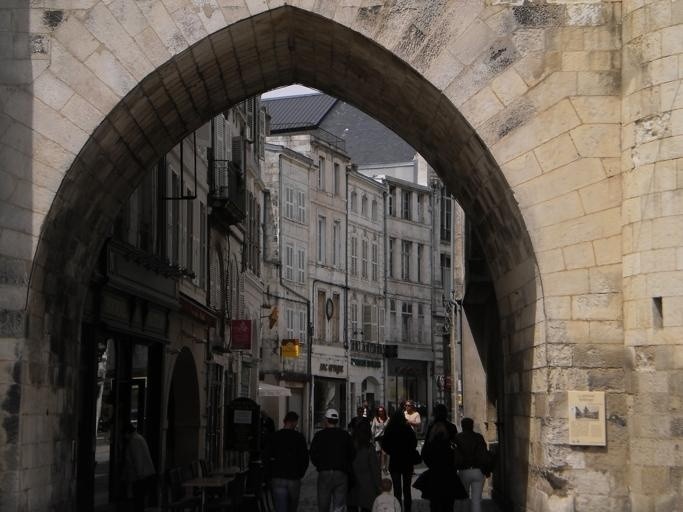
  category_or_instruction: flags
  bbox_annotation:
[229,318,253,353]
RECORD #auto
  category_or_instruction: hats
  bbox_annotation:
[322,407,339,420]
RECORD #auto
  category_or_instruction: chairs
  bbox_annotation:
[158,457,275,512]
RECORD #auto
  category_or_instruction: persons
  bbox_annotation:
[425,403,457,442]
[264,410,309,512]
[417,421,460,510]
[400,400,422,436]
[368,478,401,511]
[370,405,392,475]
[118,422,157,511]
[375,410,417,511]
[446,417,489,511]
[347,407,371,436]
[308,407,355,512]
